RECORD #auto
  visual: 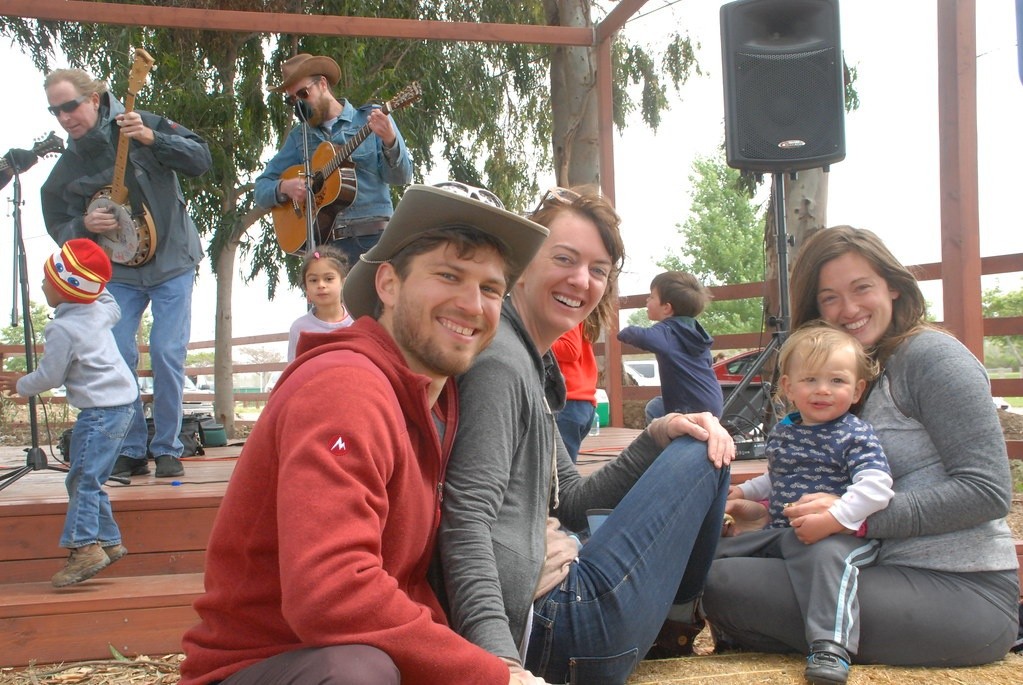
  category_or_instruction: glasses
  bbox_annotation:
[284,80,317,106]
[431,181,506,210]
[531,186,592,217]
[48,96,86,115]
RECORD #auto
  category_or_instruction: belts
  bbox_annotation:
[329,219,389,241]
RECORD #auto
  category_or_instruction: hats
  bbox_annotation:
[266,54,341,92]
[340,184,550,320]
[44,239,112,303]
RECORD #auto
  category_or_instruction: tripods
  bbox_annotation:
[0,166,131,491]
[725,173,794,441]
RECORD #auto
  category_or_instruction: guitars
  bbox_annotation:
[271,79,423,260]
[81,46,159,269]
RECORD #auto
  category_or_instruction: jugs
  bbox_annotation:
[594,388,610,427]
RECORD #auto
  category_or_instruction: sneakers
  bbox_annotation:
[111,454,151,477]
[52,541,127,588]
[154,455,184,478]
[804,640,851,685]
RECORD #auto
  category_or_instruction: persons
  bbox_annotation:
[179,183,549,685]
[428,179,737,685]
[0,237,141,587]
[38,68,212,478]
[287,245,354,370]
[252,52,412,276]
[698,225,1018,668]
[545,320,599,472]
[618,271,724,429]
[726,317,896,682]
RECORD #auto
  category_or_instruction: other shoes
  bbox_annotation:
[643,601,706,660]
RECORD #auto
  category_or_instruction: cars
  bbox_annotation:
[625,360,661,385]
[713,348,766,381]
[52,374,215,419]
[264,371,282,393]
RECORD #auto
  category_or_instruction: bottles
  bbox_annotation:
[588,411,599,436]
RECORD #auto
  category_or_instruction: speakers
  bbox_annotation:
[720,0,845,180]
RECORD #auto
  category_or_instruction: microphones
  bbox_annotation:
[295,100,314,121]
[8,148,36,170]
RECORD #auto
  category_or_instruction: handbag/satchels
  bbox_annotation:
[146,415,206,459]
